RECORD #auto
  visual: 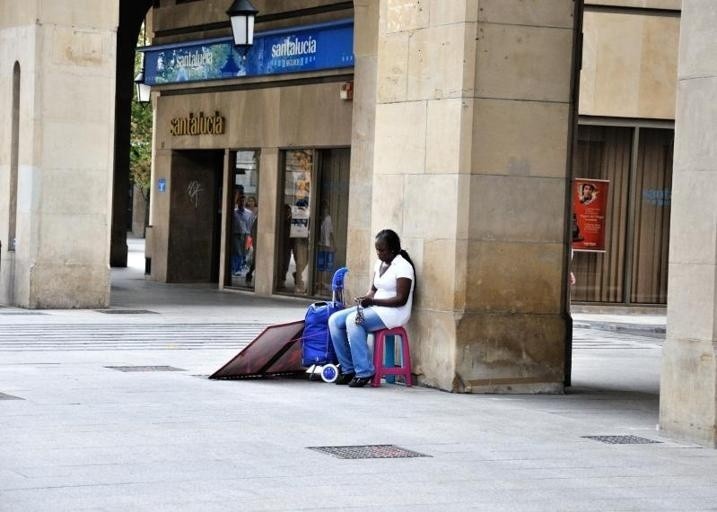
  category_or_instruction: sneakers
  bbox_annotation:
[349,372,376,387]
[335,373,355,385]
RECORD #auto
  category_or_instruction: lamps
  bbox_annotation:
[225,0,259,56]
[135,16,154,107]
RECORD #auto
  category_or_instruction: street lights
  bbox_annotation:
[135,48,160,287]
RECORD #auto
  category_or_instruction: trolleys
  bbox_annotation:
[297,264,353,385]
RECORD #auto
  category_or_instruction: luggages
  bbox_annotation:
[301,268,350,383]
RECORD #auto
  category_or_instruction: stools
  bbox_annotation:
[367,330,413,387]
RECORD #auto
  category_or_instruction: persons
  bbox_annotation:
[580,184,593,204]
[230,196,333,293]
[326,229,414,386]
